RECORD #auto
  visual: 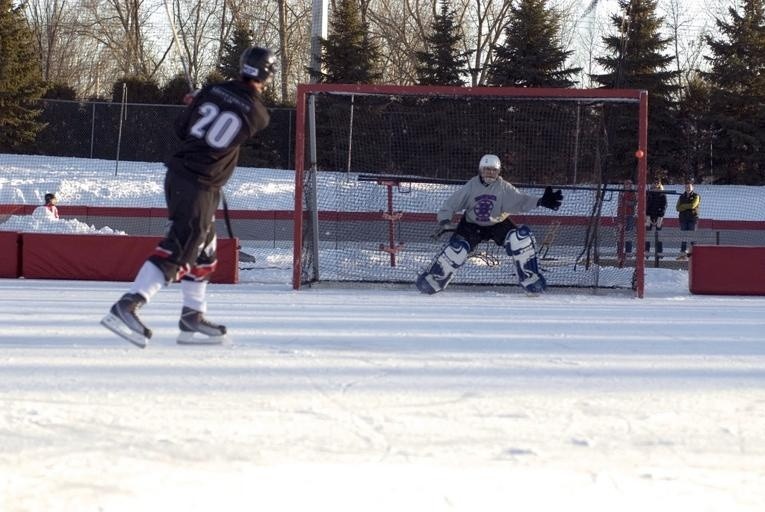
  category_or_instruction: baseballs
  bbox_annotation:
[636,150,643,159]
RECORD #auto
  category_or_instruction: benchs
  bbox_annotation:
[615,229,720,268]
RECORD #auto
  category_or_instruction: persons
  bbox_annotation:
[111,47,277,339]
[644,176,668,259]
[43,194,59,219]
[618,180,636,257]
[676,181,701,256]
[416,154,563,293]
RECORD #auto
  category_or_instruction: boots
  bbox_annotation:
[179,306,227,336]
[111,292,152,338]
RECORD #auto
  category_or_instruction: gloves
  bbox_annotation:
[536,186,563,211]
[433,219,459,240]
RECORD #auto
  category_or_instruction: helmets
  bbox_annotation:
[45,194,54,200]
[479,154,501,173]
[240,45,279,81]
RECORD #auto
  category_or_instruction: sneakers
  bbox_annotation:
[676,251,686,259]
